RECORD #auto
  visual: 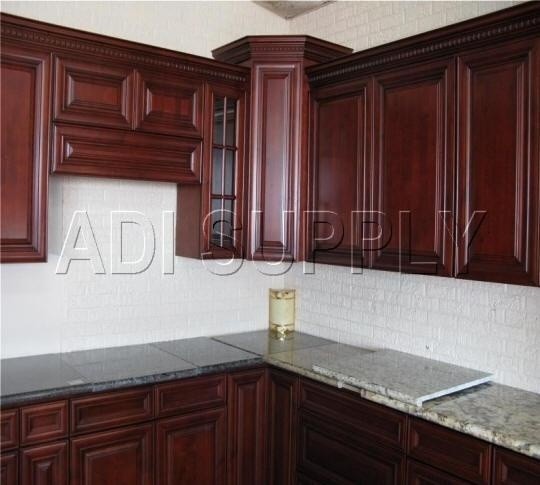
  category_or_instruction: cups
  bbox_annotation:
[269,286,295,339]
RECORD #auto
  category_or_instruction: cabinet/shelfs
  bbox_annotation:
[50,23,205,186]
[0,12,52,266]
[0,364,540,485]
[175,57,251,262]
[210,34,353,263]
[306,0,540,288]
